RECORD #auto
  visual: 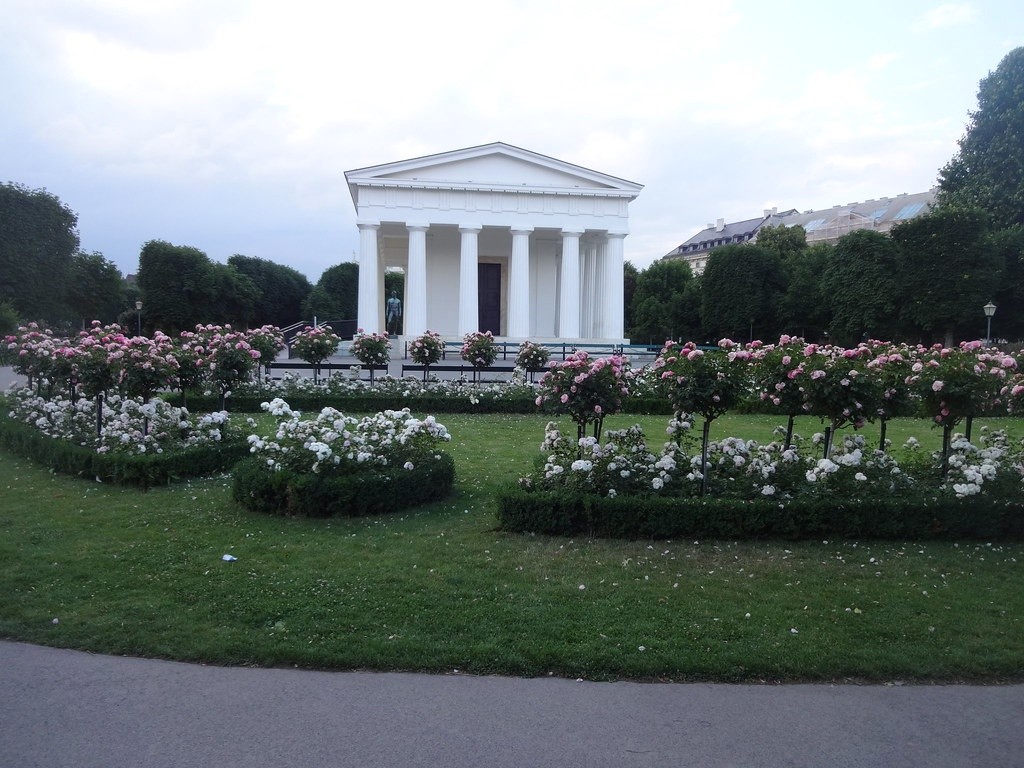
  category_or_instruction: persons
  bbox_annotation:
[386,290,401,336]
